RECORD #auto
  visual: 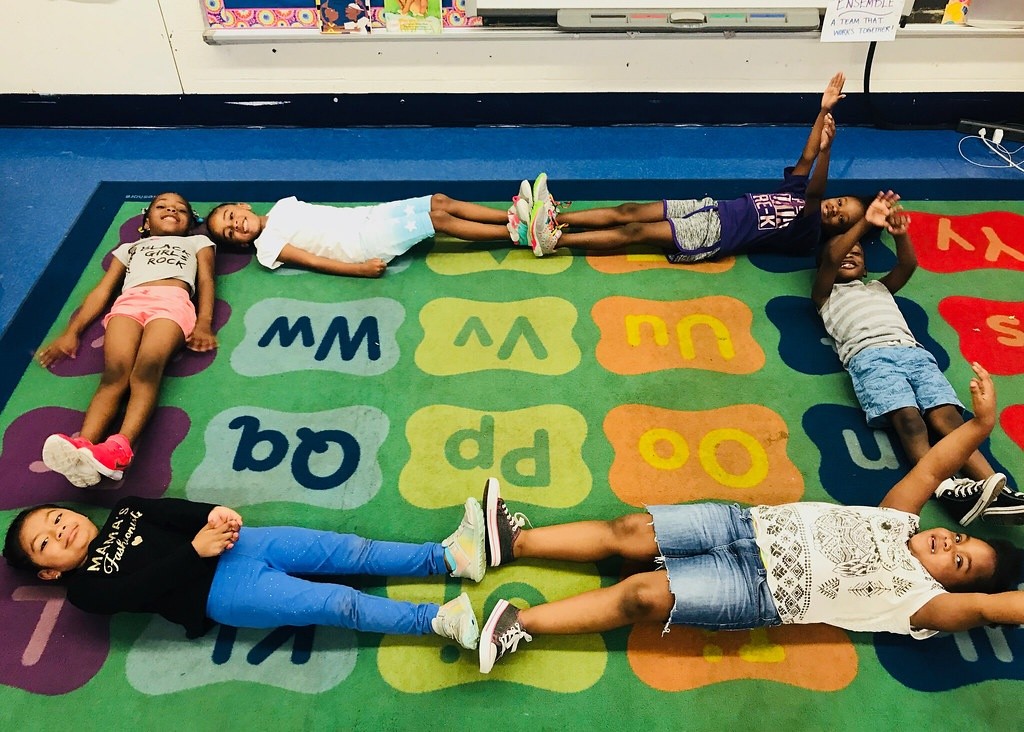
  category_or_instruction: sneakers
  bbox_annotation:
[981,486,1024,526]
[431,592,479,650]
[42,433,101,487]
[933,473,1006,526]
[508,180,533,220]
[530,202,569,256]
[77,434,133,481]
[507,199,532,246]
[483,477,533,567]
[533,173,572,218]
[442,497,486,582]
[478,600,532,674]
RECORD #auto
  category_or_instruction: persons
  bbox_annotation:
[812,190,1024,526]
[39,192,219,487]
[3,495,486,649]
[529,73,869,265]
[205,180,533,278]
[477,362,1024,674]
